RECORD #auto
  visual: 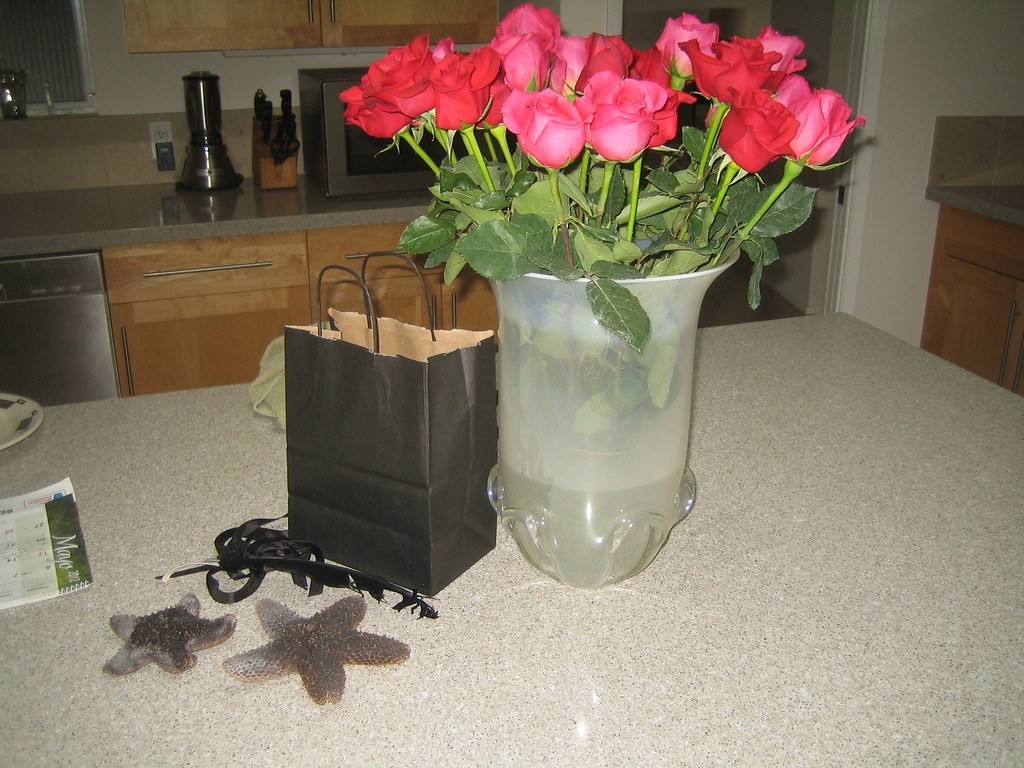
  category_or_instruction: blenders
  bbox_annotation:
[175,70,245,193]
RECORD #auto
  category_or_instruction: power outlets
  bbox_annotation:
[151,122,173,159]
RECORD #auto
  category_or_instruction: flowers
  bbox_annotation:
[339,4,864,354]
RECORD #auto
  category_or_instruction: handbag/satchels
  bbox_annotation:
[282,251,499,598]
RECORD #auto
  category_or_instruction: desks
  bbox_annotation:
[0,310,1024,768]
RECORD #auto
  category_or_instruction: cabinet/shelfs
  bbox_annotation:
[123,0,498,54]
[102,223,500,396]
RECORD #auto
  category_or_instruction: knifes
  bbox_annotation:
[253,88,296,144]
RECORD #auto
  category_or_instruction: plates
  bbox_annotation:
[0,392,45,451]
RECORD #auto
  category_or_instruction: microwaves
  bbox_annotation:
[300,67,517,197]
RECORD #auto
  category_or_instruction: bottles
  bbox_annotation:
[0,72,24,118]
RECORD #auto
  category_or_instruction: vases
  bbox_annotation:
[487,247,740,591]
